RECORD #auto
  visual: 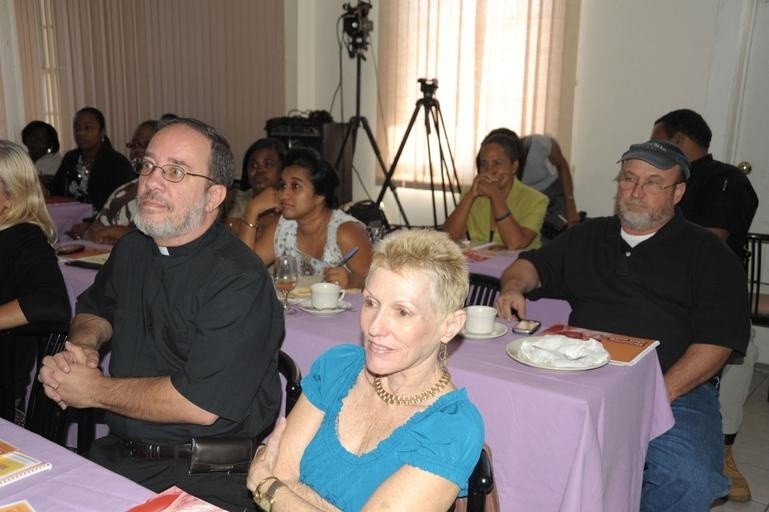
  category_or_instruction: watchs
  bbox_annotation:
[259,479,287,511]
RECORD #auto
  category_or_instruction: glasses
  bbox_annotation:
[130,155,218,185]
[618,177,677,193]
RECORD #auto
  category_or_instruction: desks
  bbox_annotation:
[44,197,94,240]
[50,238,677,512]
[0,419,160,512]
[461,247,572,327]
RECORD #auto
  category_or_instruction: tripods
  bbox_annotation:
[307,47,416,230]
[375,99,462,231]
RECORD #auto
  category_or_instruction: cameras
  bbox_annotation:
[340,0,375,42]
[418,77,436,101]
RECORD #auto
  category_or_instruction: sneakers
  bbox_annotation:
[721,444,753,503]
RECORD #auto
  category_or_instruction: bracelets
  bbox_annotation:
[495,211,512,222]
[241,218,257,228]
[253,476,278,504]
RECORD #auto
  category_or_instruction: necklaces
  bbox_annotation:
[372,369,452,406]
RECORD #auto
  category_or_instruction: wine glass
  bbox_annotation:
[271,255,295,314]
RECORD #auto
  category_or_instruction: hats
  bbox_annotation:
[616,139,691,181]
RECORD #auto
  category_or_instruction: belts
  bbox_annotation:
[109,434,190,462]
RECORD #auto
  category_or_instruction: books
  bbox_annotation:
[0,438,53,487]
[124,485,228,512]
[45,196,81,207]
[0,500,37,512]
[64,246,113,270]
[534,324,662,367]
[461,240,506,264]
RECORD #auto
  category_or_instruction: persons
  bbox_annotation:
[222,137,285,238]
[20,120,63,196]
[518,135,581,237]
[492,139,753,512]
[246,225,485,512]
[38,118,286,512]
[238,146,373,290]
[440,133,549,252]
[83,121,168,244]
[648,107,759,511]
[0,138,72,423]
[41,107,137,208]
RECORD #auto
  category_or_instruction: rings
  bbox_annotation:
[229,222,233,228]
[334,280,340,285]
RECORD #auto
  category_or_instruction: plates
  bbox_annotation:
[299,299,357,317]
[452,321,509,339]
[504,334,611,370]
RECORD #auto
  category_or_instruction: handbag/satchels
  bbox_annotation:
[348,199,390,240]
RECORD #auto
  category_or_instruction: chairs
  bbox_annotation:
[463,273,499,309]
[745,233,769,330]
[278,350,304,418]
[25,322,74,447]
[450,449,494,512]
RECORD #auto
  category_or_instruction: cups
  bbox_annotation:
[463,304,498,334]
[313,282,347,307]
